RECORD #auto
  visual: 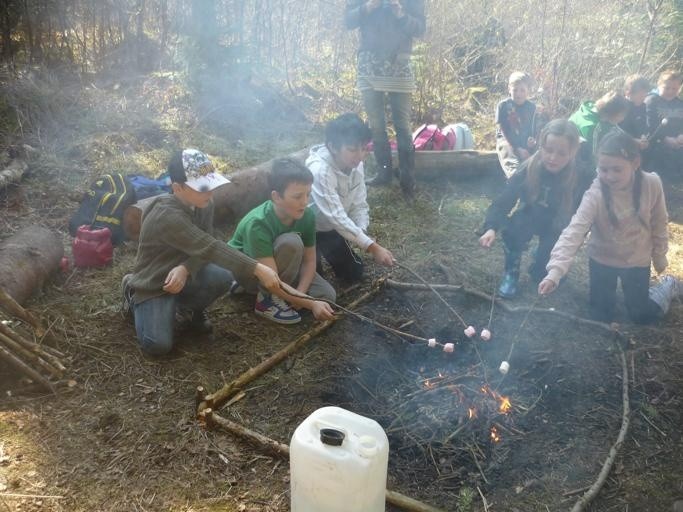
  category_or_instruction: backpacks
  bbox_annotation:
[413,124,456,150]
[452,123,473,150]
[69,174,137,243]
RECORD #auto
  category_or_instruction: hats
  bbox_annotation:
[168,148,231,192]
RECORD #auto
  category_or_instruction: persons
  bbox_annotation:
[568,88,629,148]
[478,118,597,301]
[493,70,540,179]
[301,110,397,282]
[621,73,649,168]
[343,0,427,208]
[534,129,682,327]
[119,146,282,357]
[224,154,338,326]
[644,69,682,178]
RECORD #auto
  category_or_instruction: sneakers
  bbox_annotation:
[255,291,301,324]
[121,274,135,319]
[230,280,244,293]
[365,171,392,187]
[400,175,417,201]
[176,303,213,332]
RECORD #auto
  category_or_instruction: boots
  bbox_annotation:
[499,243,521,300]
[649,274,683,315]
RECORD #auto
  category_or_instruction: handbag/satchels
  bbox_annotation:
[72,224,113,268]
